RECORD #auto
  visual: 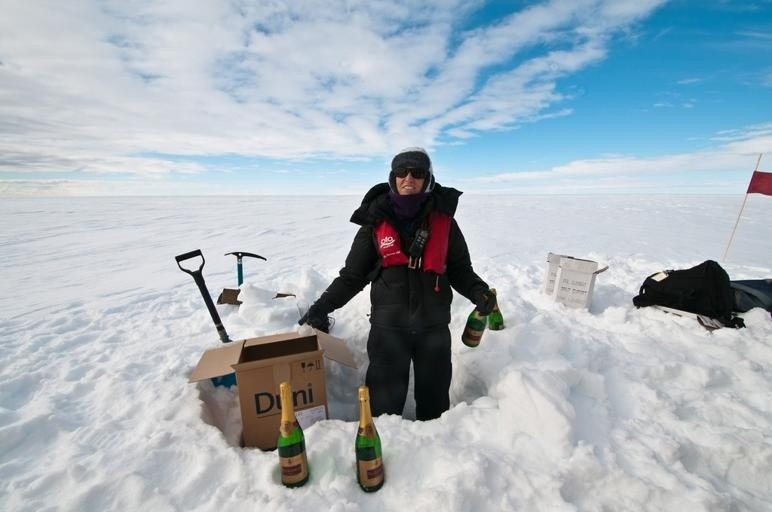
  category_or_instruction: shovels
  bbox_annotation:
[175,249,236,388]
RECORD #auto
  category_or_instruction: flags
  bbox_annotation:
[747,170,772,196]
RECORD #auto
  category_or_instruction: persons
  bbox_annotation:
[299,146,497,422]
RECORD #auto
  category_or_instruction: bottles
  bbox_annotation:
[462,289,511,348]
[355,384,386,493]
[276,382,310,494]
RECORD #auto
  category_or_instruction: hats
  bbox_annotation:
[389,146,435,193]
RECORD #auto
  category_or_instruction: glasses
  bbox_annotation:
[393,167,426,179]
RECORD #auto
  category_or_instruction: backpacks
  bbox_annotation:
[633,260,733,322]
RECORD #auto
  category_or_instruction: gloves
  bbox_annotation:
[471,285,494,317]
[299,306,331,333]
